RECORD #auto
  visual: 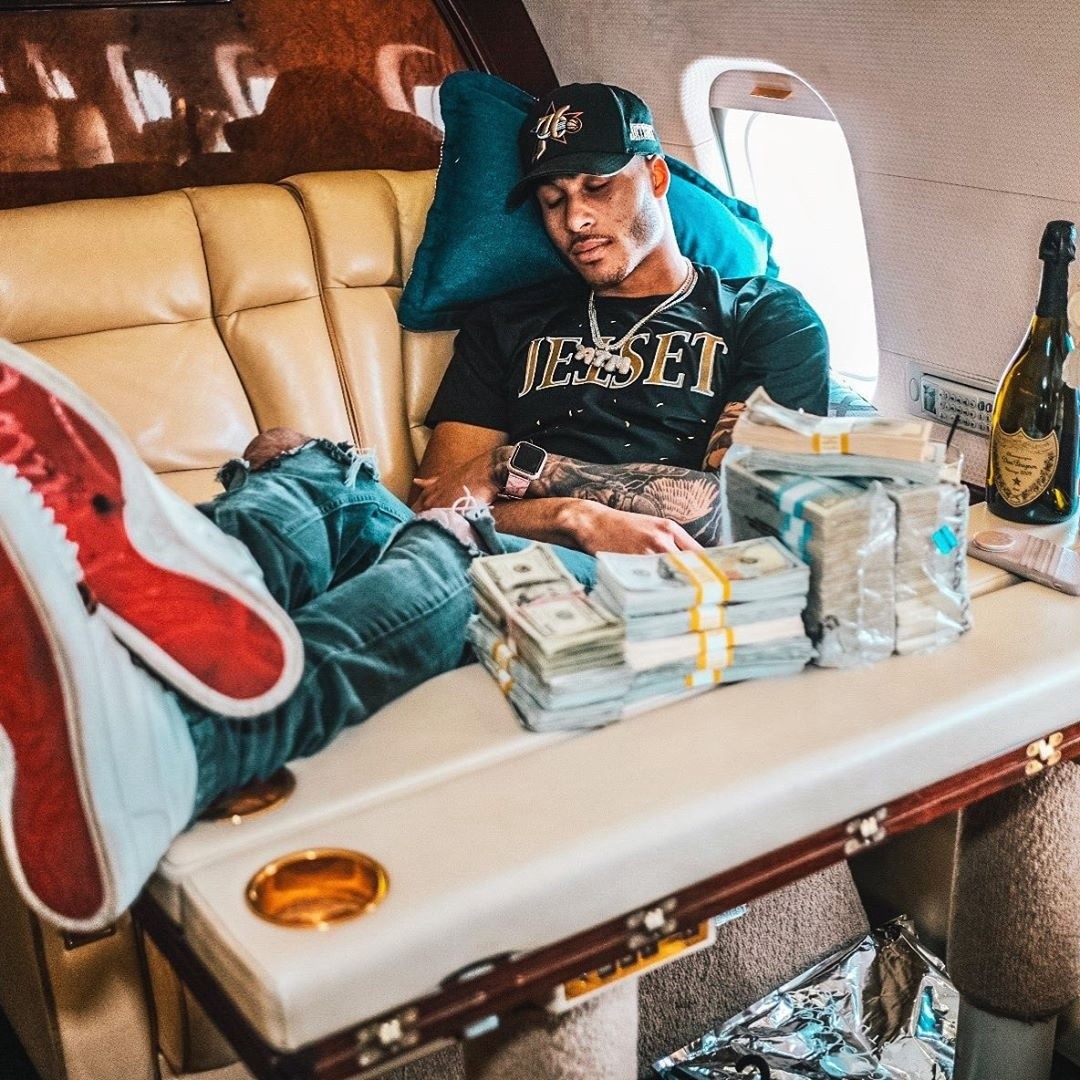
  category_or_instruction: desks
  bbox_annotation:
[129,500,1080,1080]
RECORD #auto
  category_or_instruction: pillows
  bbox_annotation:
[397,72,779,333]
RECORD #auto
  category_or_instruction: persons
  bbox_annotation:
[2,83,828,931]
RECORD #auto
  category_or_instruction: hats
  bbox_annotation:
[510,83,664,208]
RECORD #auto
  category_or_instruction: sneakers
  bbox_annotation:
[0,339,306,720]
[0,468,199,932]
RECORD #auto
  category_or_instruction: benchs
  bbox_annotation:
[0,96,461,1080]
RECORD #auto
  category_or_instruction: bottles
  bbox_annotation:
[982,216,1080,527]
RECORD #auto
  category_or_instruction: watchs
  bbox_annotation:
[497,441,547,501]
